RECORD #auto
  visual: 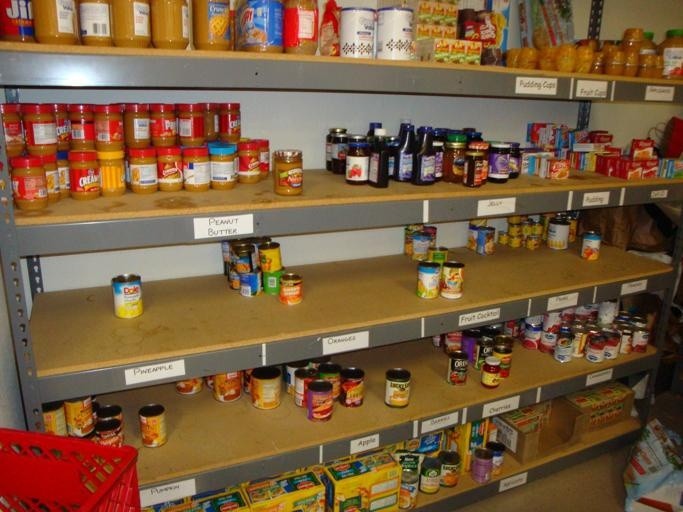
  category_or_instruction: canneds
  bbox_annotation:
[439,261,464,300]
[42,394,125,447]
[0,0,415,62]
[138,403,168,448]
[325,127,401,185]
[221,237,304,306]
[416,260,441,299]
[404,222,448,263]
[274,149,302,198]
[467,210,579,256]
[175,355,365,422]
[581,228,603,259]
[433,303,650,389]
[0,102,269,212]
[384,367,411,408]
[432,134,521,188]
[111,274,144,319]
[395,441,506,509]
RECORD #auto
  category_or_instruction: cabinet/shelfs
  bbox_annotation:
[0,37,681,512]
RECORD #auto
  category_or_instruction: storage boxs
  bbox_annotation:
[497,395,583,466]
[241,444,402,512]
[565,378,633,438]
[142,484,247,511]
[392,417,490,478]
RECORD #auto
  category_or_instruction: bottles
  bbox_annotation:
[642,28,682,79]
[364,121,387,186]
[398,122,435,185]
